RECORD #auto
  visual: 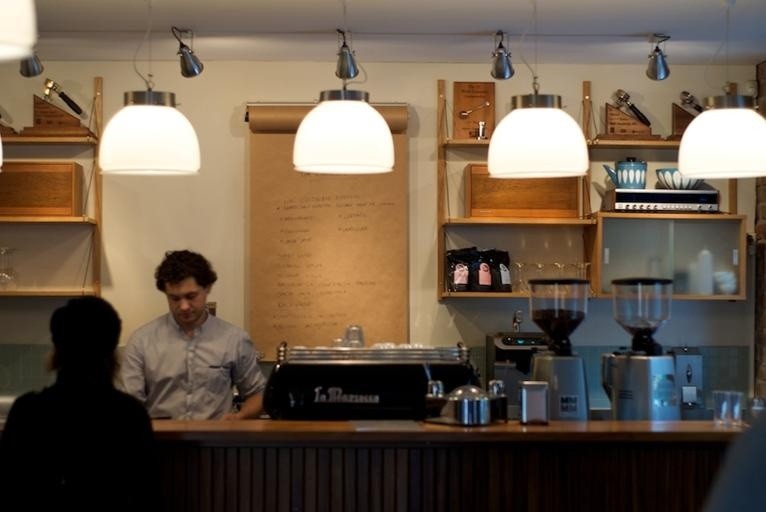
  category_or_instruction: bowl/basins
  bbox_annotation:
[714,271,737,294]
[656,167,704,190]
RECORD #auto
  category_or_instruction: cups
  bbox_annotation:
[711,389,766,430]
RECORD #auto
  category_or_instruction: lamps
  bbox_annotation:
[646,33,671,81]
[491,29,514,80]
[97,87,203,175]
[677,94,766,179]
[20,57,43,77]
[485,94,590,181]
[334,28,359,79]
[291,90,396,175]
[171,26,203,78]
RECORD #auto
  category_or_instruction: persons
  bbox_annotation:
[115,250,267,421]
[0,295,200,512]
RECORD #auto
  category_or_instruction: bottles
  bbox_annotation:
[695,249,714,297]
[517,380,551,427]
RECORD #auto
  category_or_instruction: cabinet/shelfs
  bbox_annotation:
[436,80,748,302]
[0,76,102,297]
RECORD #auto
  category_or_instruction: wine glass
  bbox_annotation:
[512,262,592,295]
[0,248,16,289]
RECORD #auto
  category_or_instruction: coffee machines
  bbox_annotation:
[528,279,591,421]
[601,277,681,421]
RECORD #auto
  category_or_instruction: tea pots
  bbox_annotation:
[602,156,647,189]
